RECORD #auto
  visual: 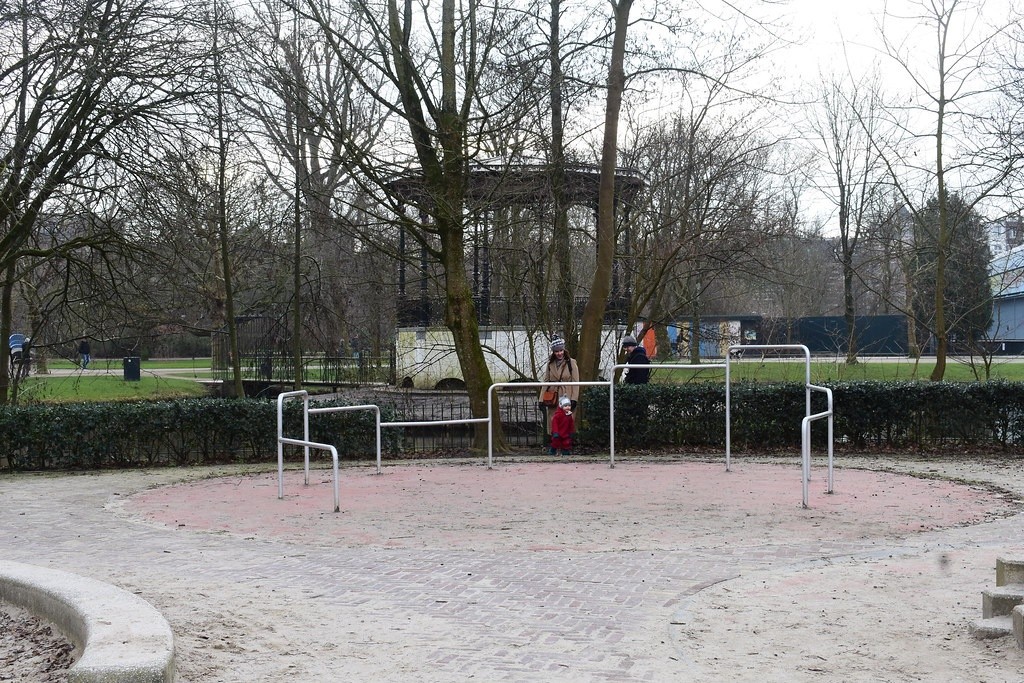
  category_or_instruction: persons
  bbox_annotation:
[79,336,91,369]
[21,338,31,377]
[351,333,361,366]
[676,330,691,352]
[550,396,575,455]
[539,339,579,454]
[621,335,649,384]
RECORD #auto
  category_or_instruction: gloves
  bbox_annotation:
[540,403,545,411]
[571,401,576,412]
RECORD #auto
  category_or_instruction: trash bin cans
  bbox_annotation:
[123,356,141,381]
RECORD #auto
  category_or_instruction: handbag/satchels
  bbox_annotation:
[543,392,558,406]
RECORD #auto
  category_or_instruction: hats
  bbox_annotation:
[622,332,637,345]
[559,397,571,408]
[550,334,565,351]
